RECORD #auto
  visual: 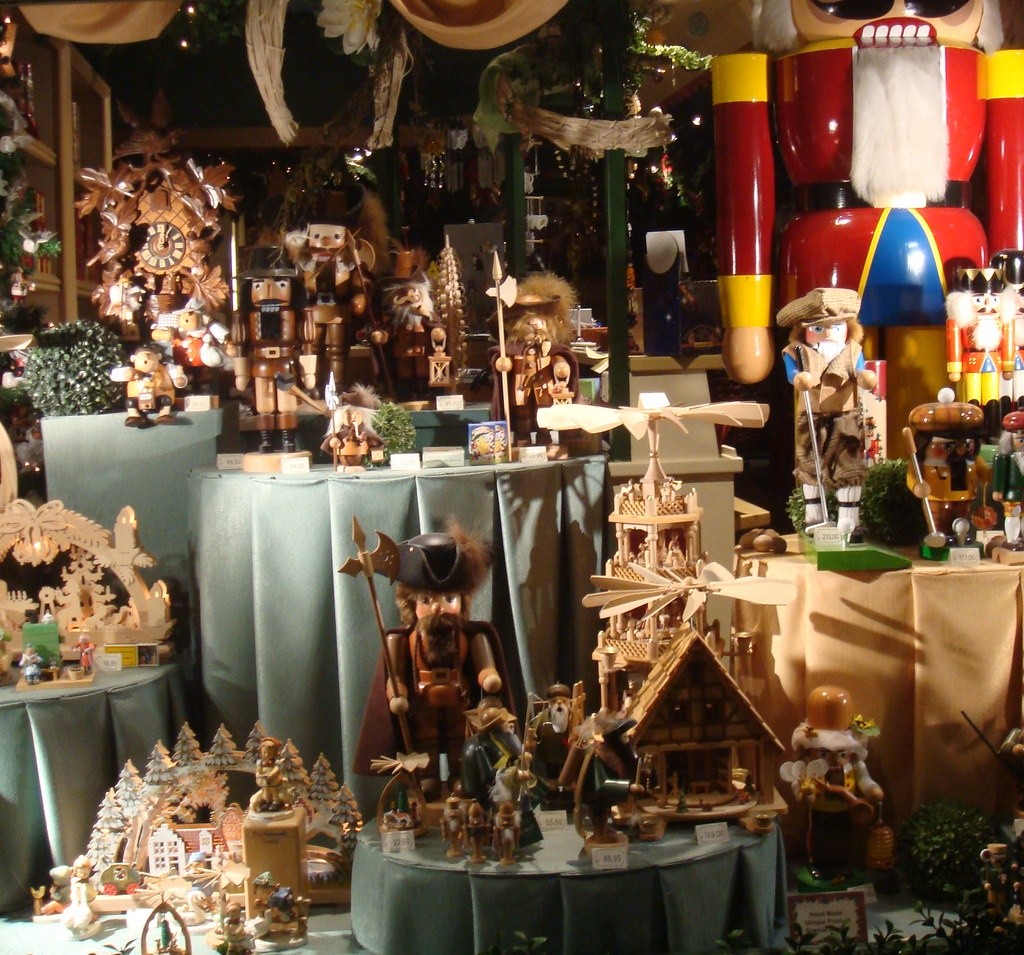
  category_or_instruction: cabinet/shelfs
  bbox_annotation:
[2,17,117,334]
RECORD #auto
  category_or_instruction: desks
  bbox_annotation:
[189,455,608,801]
[0,659,366,954]
[730,533,1024,841]
[354,797,788,955]
